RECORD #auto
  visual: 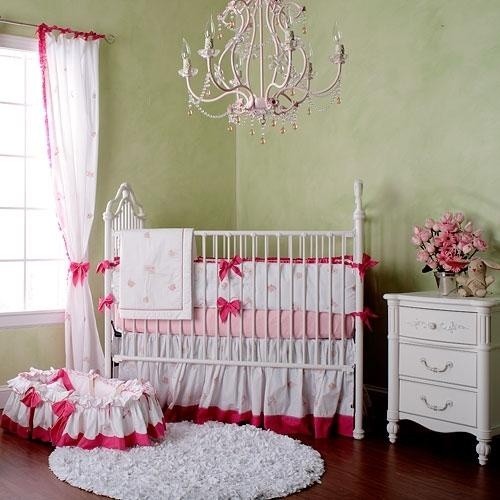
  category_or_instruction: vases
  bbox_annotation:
[437,271,457,296]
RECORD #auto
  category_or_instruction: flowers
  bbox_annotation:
[411,210,488,275]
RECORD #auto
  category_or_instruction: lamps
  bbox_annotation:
[179,0,346,145]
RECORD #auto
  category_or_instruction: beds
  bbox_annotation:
[102,182,366,439]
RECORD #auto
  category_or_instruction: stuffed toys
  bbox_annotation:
[457,256,500,297]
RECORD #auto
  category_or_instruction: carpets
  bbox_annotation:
[48,420,325,499]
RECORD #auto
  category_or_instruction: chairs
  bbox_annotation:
[382,290,500,466]
